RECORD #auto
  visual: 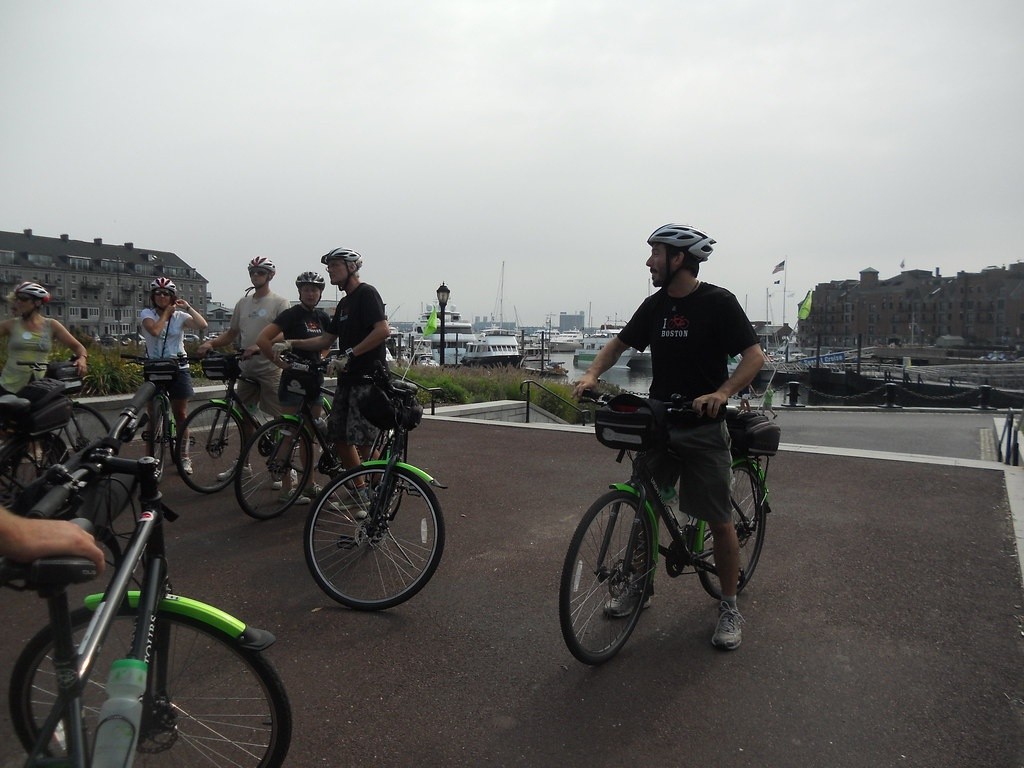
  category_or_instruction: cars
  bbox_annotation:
[92,331,219,346]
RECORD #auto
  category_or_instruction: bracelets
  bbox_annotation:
[80,355,87,359]
[185,305,190,311]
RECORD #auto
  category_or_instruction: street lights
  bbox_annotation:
[436,281,451,372]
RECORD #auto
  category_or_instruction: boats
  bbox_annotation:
[386,296,650,379]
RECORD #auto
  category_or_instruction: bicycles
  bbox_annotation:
[559,389,772,668]
[0,347,450,768]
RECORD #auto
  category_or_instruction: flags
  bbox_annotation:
[772,261,785,274]
[798,289,812,320]
[774,280,780,284]
[425,309,437,337]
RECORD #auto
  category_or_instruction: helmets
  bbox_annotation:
[14,282,49,302]
[248,256,275,274]
[150,277,176,294]
[296,271,325,291]
[648,222,717,263]
[321,247,362,268]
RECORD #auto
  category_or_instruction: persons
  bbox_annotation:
[255,271,334,503]
[197,257,297,488]
[141,277,208,473]
[571,224,765,649]
[272,248,391,510]
[0,505,105,581]
[741,383,777,419]
[276,271,277,272]
[0,282,87,485]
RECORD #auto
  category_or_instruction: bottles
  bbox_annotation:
[247,404,267,426]
[91,654,148,768]
[660,486,689,528]
[166,408,175,437]
[315,417,329,438]
[36,447,43,467]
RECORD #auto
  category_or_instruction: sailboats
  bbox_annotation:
[478,260,517,335]
[740,288,808,363]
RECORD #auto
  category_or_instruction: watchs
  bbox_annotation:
[346,347,356,360]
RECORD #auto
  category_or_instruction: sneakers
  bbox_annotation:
[217,460,253,480]
[712,600,745,650]
[604,589,651,616]
[182,458,194,473]
[272,469,298,489]
[330,487,372,509]
[279,488,310,505]
[302,484,336,498]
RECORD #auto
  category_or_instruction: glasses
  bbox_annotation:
[15,296,35,301]
[249,269,270,276]
[153,290,171,295]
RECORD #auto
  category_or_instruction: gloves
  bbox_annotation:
[272,341,290,360]
[327,356,353,379]
